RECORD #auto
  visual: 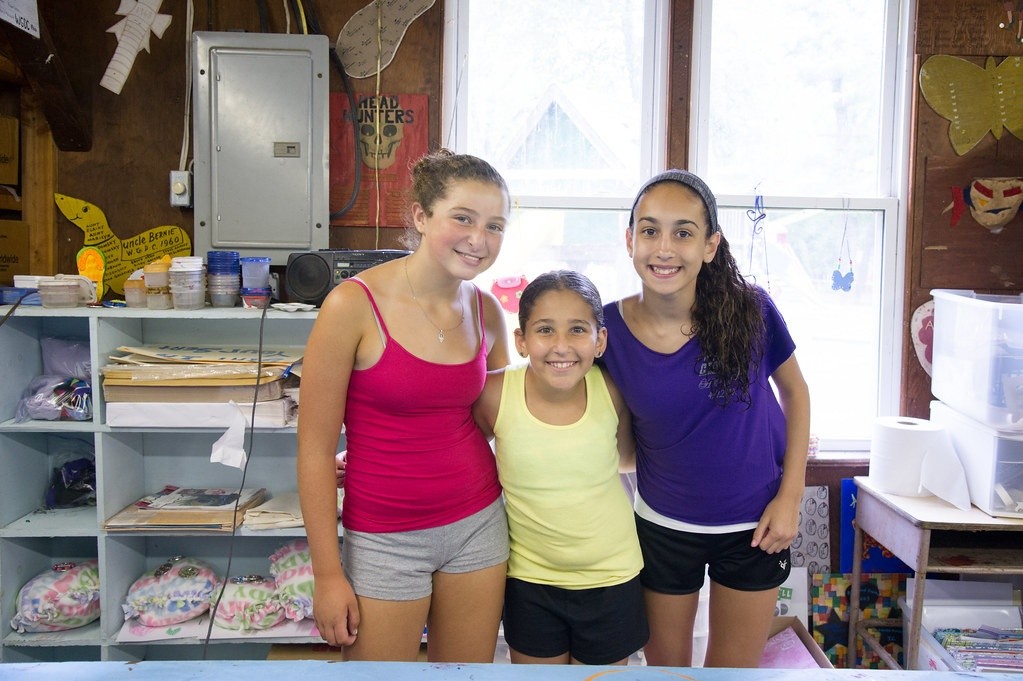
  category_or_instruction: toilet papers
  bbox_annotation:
[868,415,977,517]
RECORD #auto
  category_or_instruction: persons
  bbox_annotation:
[599,169,811,667]
[334,269,649,665]
[305,161,510,663]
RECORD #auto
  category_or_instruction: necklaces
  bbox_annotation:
[403,256,466,343]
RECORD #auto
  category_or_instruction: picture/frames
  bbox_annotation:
[324,90,431,229]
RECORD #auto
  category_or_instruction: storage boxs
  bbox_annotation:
[922,286,1022,434]
[916,397,1023,522]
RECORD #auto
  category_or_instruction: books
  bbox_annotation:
[100,343,343,531]
[761,628,825,668]
[923,617,1023,673]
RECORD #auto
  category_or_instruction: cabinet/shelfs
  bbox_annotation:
[2,292,348,660]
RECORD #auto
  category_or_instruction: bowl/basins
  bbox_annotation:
[1,250,273,311]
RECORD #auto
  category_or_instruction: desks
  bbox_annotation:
[844,474,1023,672]
